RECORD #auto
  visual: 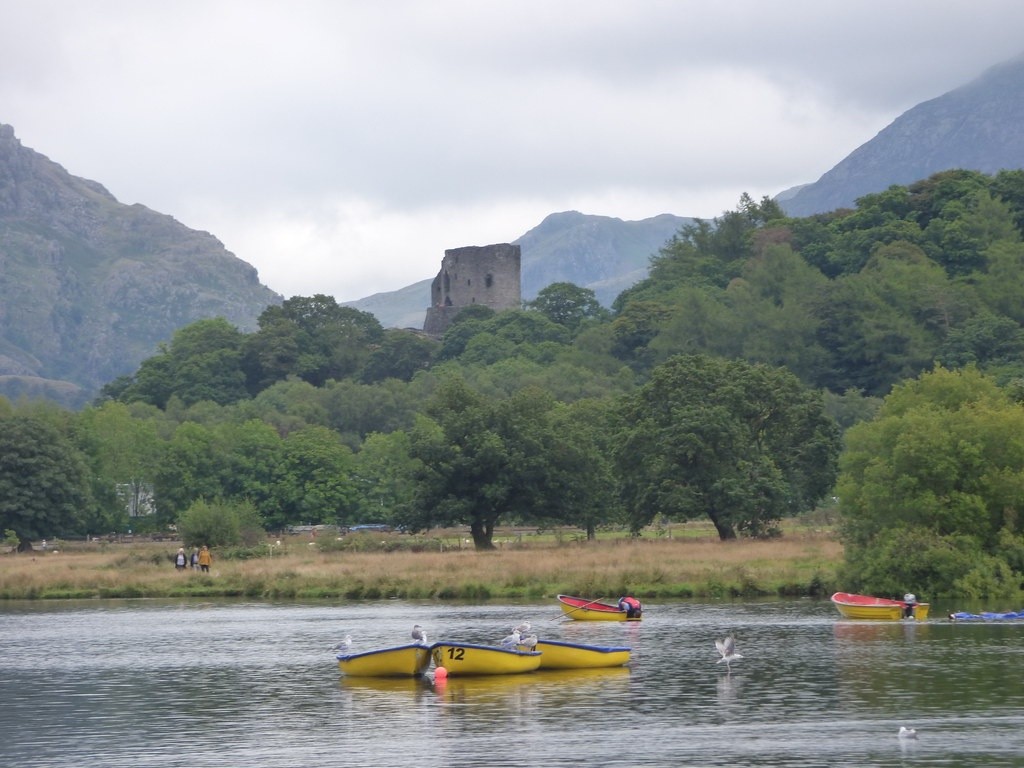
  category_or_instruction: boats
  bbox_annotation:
[432,634,542,674]
[325,623,433,678]
[830,591,930,620]
[556,593,645,622]
[515,636,633,668]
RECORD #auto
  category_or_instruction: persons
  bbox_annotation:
[191,545,212,573]
[41,540,46,548]
[311,527,317,542]
[174,548,188,570]
[617,596,642,618]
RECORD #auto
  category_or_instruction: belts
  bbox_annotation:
[194,563,198,564]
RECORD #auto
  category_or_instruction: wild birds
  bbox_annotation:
[898,726,918,739]
[411,624,427,644]
[512,619,532,639]
[515,634,538,652]
[325,634,352,656]
[500,630,523,649]
[713,632,744,670]
